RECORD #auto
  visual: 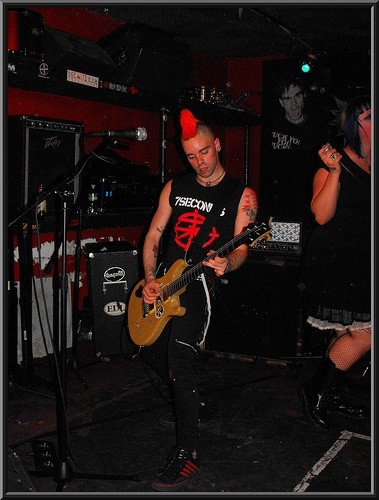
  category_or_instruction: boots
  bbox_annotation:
[325,382,369,419]
[297,358,348,432]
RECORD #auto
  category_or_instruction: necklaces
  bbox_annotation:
[197,170,224,188]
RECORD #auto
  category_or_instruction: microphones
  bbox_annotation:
[90,127,147,141]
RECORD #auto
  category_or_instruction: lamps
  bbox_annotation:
[296,47,312,73]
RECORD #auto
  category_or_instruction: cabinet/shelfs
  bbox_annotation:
[6,52,264,234]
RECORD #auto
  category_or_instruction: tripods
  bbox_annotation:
[6,141,153,492]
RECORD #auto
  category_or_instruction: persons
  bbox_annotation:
[140,110,258,491]
[293,95,371,433]
[267,72,328,225]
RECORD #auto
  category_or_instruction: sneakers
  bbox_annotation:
[152,446,200,492]
[159,408,176,428]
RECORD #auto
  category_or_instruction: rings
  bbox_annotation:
[331,154,337,160]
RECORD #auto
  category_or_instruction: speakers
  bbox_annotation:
[7,113,85,232]
[205,246,309,363]
[84,241,139,357]
[44,26,117,80]
[96,20,195,98]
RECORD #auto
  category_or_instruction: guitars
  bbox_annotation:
[127,222,272,347]
